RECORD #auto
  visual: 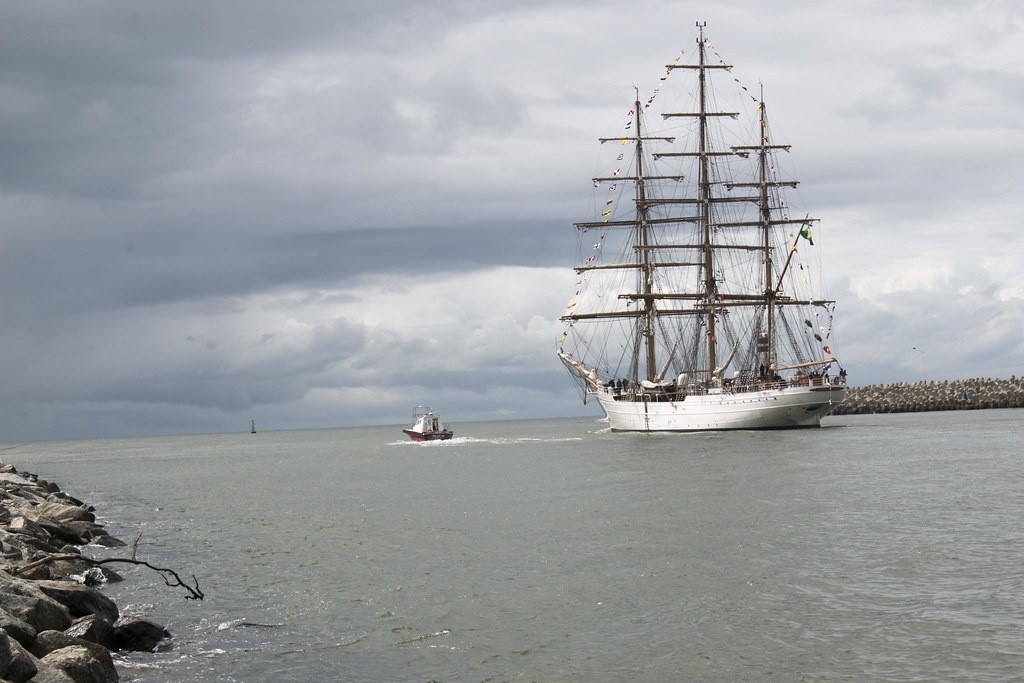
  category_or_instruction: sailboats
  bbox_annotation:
[249,420,256,434]
[554,18,849,432]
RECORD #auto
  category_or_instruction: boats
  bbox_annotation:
[402,412,455,442]
